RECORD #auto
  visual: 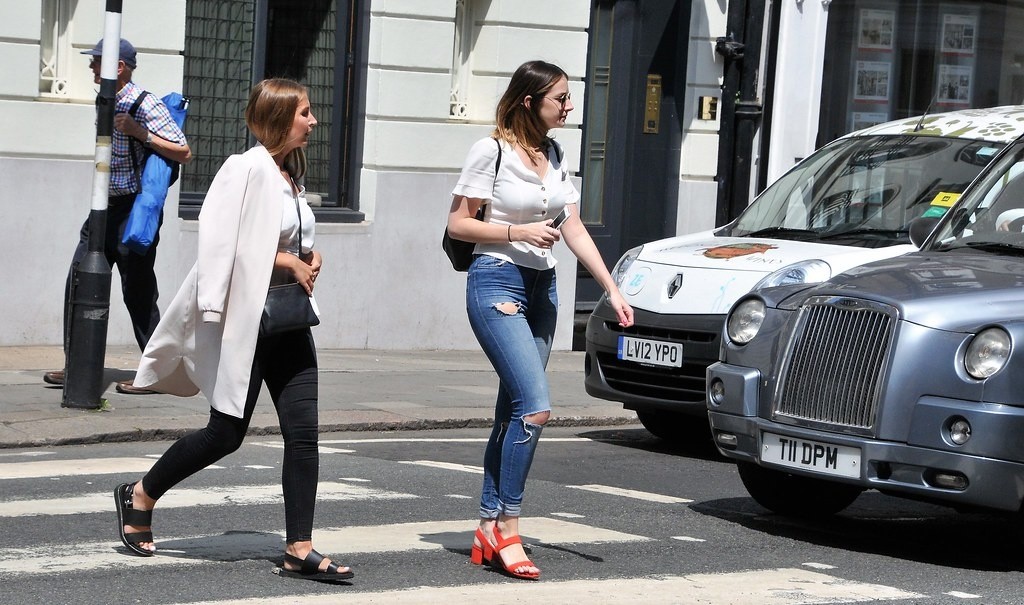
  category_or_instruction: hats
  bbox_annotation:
[80,36,137,69]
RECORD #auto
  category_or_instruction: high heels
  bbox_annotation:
[484,527,540,579]
[471,527,498,565]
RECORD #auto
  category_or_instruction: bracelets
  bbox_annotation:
[508,225,512,242]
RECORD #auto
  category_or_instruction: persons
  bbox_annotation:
[448,61,635,578]
[114,78,355,582]
[42,39,192,395]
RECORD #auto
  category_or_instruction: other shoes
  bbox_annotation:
[116,379,162,394]
[44,369,65,384]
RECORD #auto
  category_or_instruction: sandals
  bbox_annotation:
[279,549,354,580]
[114,481,155,556]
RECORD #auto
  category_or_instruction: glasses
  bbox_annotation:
[542,93,571,107]
[89,57,101,65]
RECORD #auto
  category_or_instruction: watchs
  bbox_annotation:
[142,130,153,147]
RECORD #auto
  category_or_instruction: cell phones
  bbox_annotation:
[548,205,570,229]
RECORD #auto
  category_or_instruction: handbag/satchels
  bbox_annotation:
[258,279,320,337]
[321,339,327,342]
[443,137,501,273]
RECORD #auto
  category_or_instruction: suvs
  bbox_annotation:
[706,130,1023,540]
[582,102,1023,455]
